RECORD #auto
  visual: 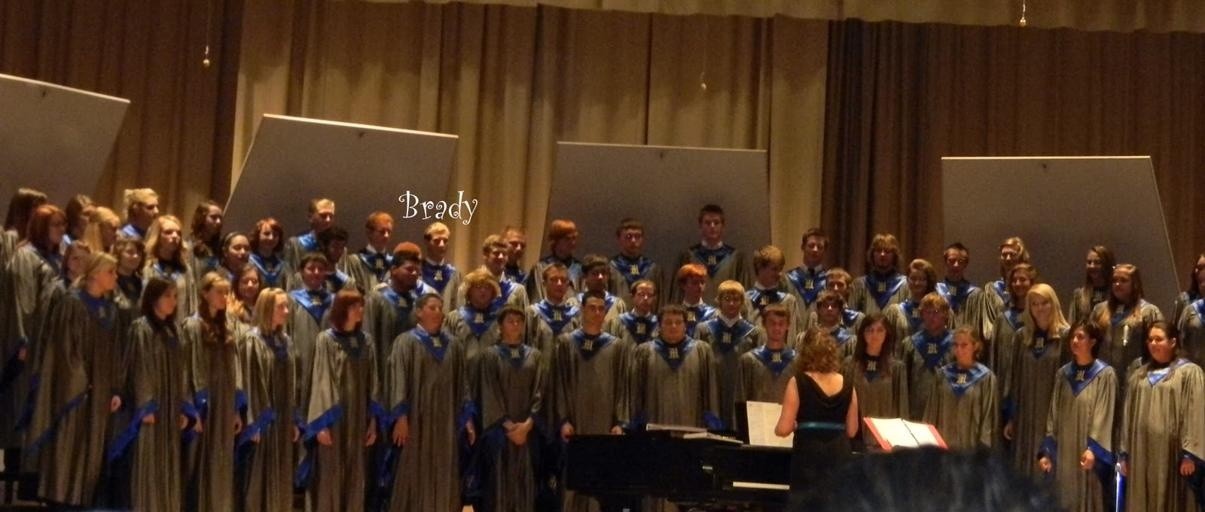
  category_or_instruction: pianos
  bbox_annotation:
[564,434,940,511]
